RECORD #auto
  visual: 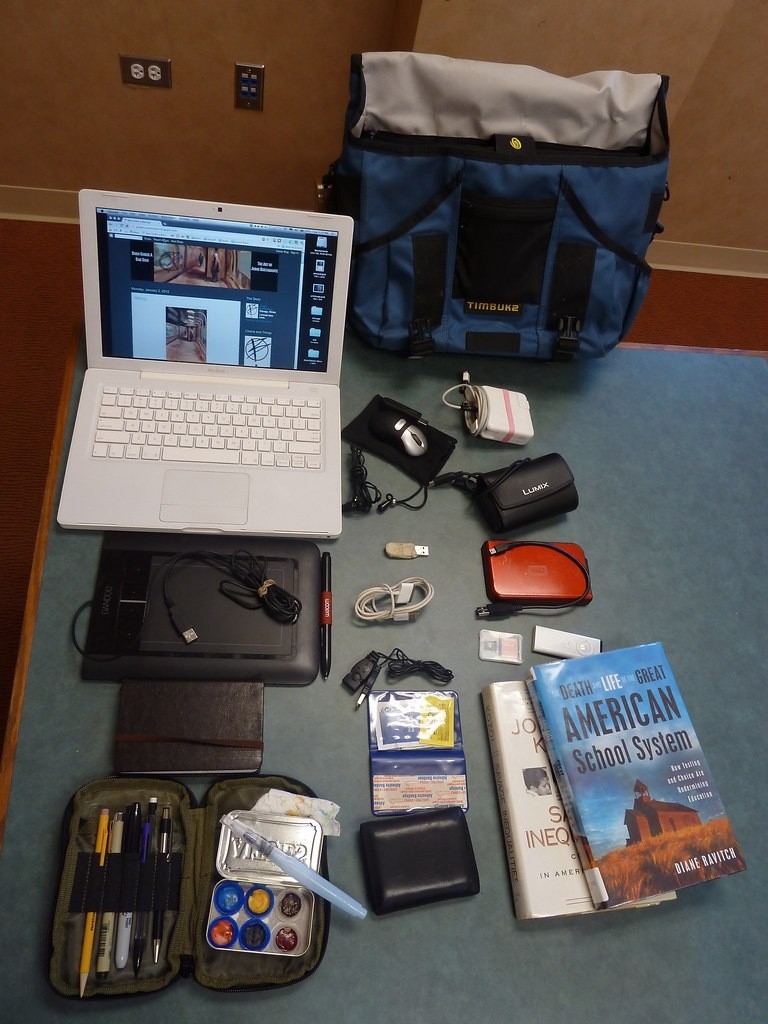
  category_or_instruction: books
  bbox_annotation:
[525,639,749,911]
[481,679,677,921]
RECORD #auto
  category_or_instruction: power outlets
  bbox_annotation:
[234,62,265,111]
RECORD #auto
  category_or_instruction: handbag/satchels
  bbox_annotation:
[321,51,670,360]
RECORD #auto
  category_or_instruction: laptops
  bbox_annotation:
[56,188,355,539]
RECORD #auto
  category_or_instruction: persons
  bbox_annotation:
[198,252,204,267]
[211,248,221,282]
[523,768,550,797]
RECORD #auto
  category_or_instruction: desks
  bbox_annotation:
[0,342,768,1024]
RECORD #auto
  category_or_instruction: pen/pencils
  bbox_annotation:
[79,808,109,998]
[96,811,124,986]
[115,801,142,971]
[132,798,158,979]
[321,551,333,681]
[152,807,172,965]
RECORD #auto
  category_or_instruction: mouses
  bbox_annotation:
[368,409,428,456]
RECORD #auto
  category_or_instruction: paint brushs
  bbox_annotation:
[218,814,368,920]
